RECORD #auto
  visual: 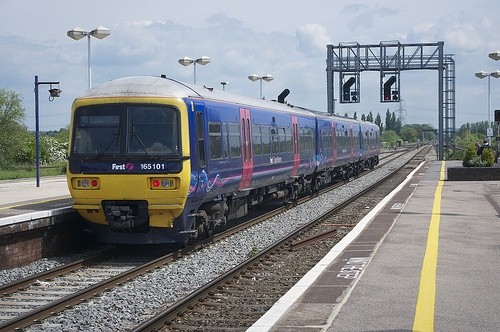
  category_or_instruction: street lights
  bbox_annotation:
[475,70,500,144]
[66,25,112,90]
[33,76,62,187]
[178,56,212,85]
[248,73,274,100]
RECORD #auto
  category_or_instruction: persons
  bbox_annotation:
[475,138,491,155]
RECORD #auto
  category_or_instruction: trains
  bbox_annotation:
[65,75,382,250]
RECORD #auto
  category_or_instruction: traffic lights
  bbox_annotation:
[384,85,391,101]
